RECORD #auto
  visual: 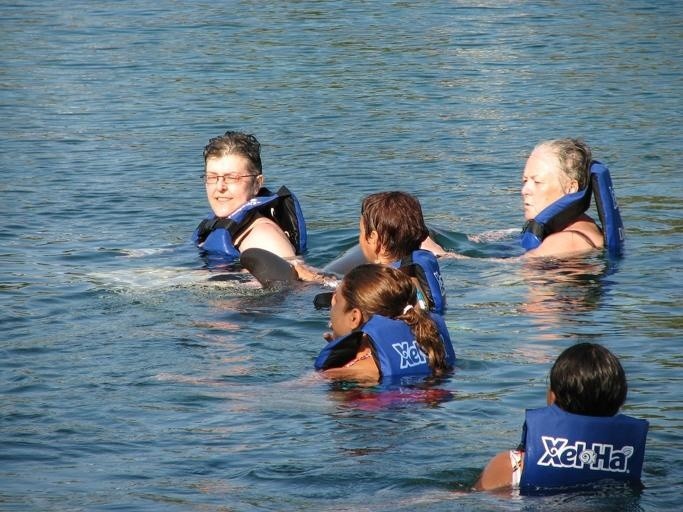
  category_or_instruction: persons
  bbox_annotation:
[420,137,625,261]
[191,130,307,287]
[288,191,446,318]
[472,342,650,491]
[298,264,456,409]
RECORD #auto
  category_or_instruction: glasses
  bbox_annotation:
[199,173,258,184]
